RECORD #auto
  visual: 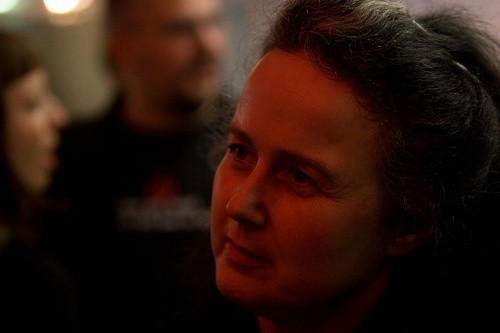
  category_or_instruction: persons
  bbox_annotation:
[0,0,239,333]
[211,0,500,333]
